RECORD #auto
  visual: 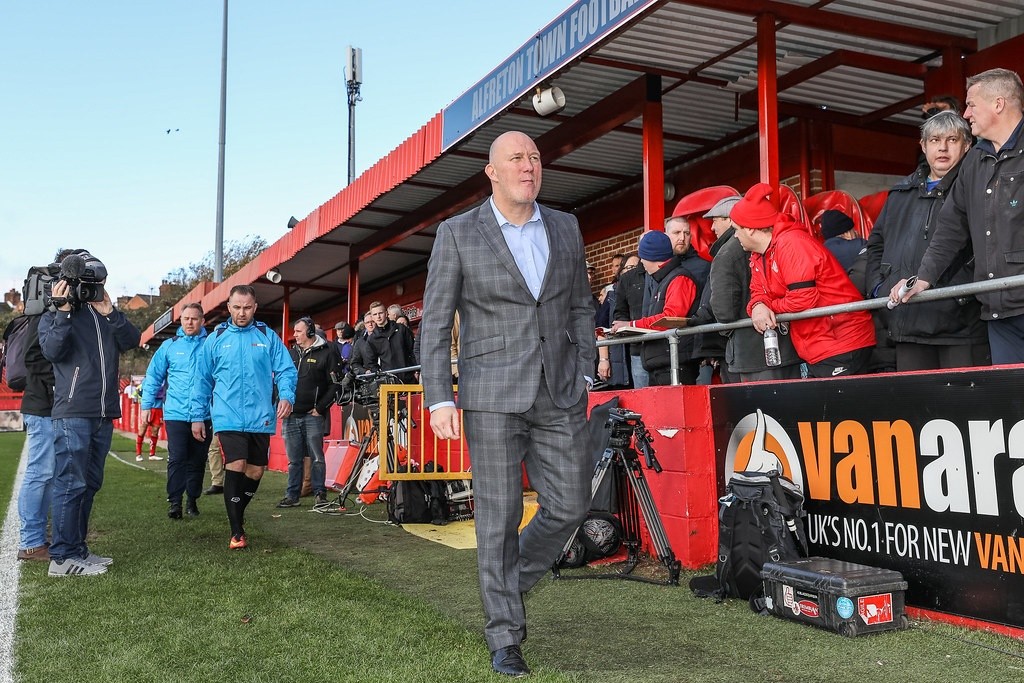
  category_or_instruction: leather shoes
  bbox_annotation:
[491,644,531,678]
[18,542,52,562]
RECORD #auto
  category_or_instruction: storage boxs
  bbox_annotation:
[759,556,912,640]
[316,439,362,493]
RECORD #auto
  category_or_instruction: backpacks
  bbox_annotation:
[3,315,31,393]
[386,466,433,524]
[712,471,809,611]
[425,460,451,524]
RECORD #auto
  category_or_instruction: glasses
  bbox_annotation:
[586,261,639,277]
[364,321,375,324]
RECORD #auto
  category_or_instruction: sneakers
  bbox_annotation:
[185,502,200,515]
[136,455,143,461]
[314,491,328,506]
[276,495,302,508]
[168,504,182,519]
[148,456,164,460]
[229,524,248,550]
[83,552,113,566]
[47,557,109,577]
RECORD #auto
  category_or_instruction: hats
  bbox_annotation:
[341,326,355,339]
[333,321,347,329]
[638,182,854,262]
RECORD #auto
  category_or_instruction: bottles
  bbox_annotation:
[763,324,782,367]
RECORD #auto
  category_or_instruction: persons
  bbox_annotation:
[664,216,712,299]
[141,302,210,519]
[612,230,702,386]
[39,248,143,576]
[202,425,227,497]
[134,368,167,462]
[728,184,876,378]
[864,111,990,372]
[818,208,866,278]
[124,294,471,426]
[14,249,80,562]
[295,401,332,498]
[847,237,896,375]
[190,285,298,549]
[888,67,1024,367]
[418,130,597,678]
[686,255,741,385]
[276,316,339,508]
[582,228,659,390]
[700,193,809,382]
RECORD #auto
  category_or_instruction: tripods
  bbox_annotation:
[338,422,410,505]
[558,450,680,587]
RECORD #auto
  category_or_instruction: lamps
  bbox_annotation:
[530,82,566,117]
[266,269,282,285]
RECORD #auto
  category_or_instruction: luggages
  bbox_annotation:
[760,555,910,638]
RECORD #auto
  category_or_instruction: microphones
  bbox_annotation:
[61,254,84,279]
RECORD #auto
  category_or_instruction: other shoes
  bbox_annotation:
[204,485,224,495]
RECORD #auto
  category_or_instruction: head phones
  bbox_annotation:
[297,319,315,336]
